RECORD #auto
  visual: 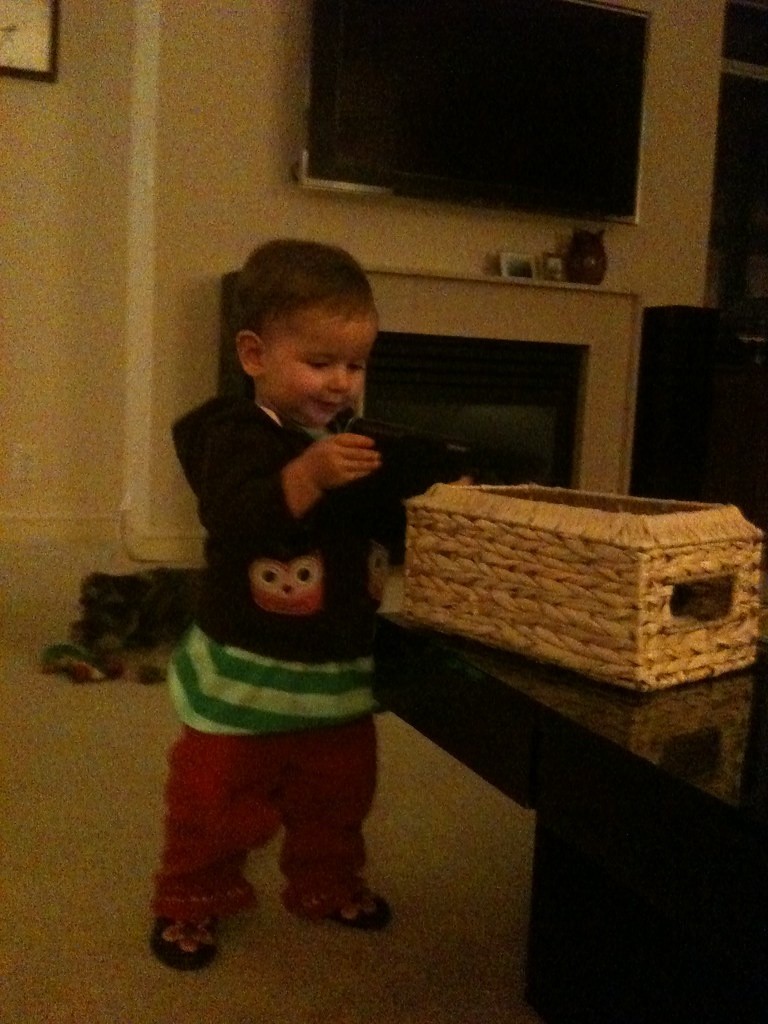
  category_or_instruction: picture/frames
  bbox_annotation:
[543,252,567,284]
[496,249,540,282]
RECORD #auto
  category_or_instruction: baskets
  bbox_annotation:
[400,480,766,694]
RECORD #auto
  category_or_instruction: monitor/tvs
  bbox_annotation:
[297,0,652,224]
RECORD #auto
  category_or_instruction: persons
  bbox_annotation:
[145,239,472,970]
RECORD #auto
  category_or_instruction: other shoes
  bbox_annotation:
[152,914,216,971]
[326,888,392,931]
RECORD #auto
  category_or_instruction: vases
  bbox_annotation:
[568,226,609,286]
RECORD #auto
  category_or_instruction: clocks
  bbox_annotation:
[0,0,62,86]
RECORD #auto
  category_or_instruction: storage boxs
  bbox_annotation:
[452,643,753,808]
[401,482,766,690]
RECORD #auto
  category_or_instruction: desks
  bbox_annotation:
[371,612,767,1024]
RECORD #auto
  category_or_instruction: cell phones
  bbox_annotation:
[341,417,467,494]
